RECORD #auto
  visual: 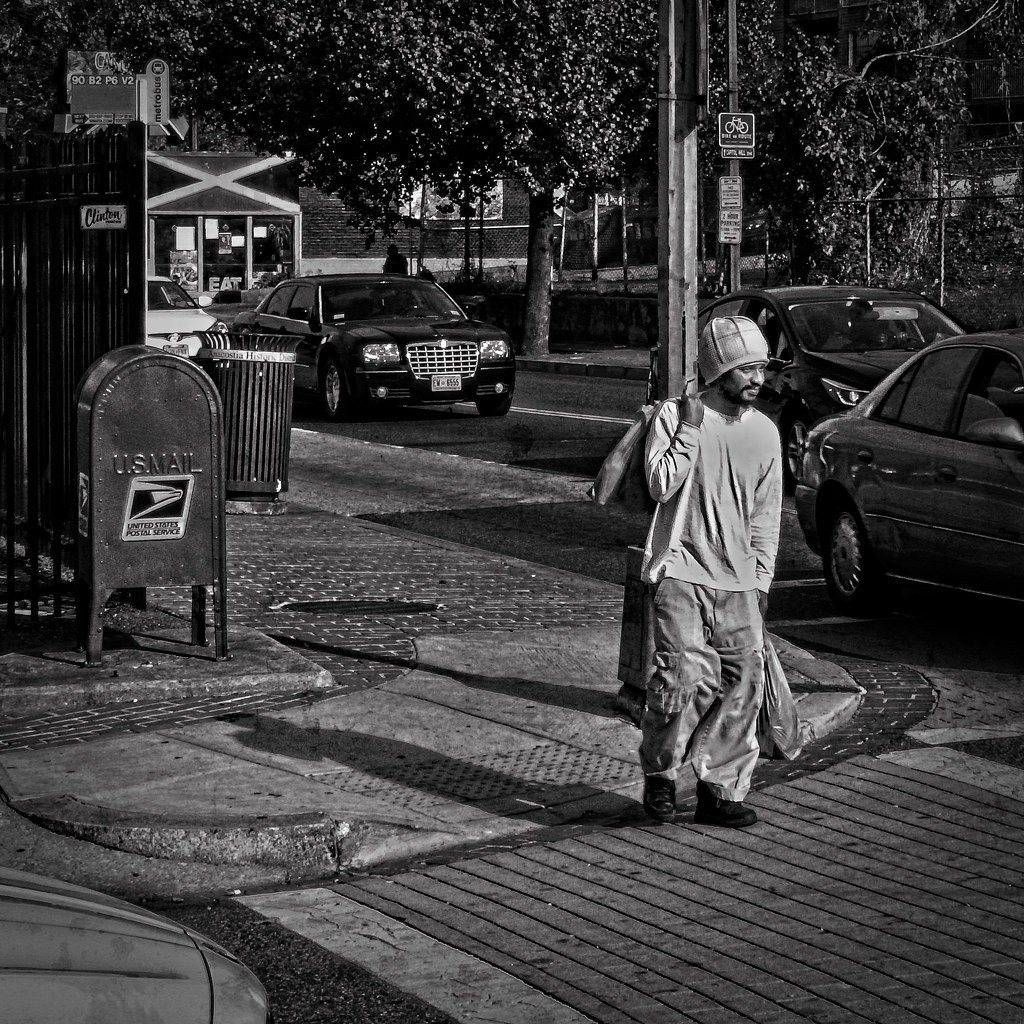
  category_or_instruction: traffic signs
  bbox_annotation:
[717,176,745,245]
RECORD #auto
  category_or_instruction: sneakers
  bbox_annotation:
[641,777,677,821]
[693,797,757,826]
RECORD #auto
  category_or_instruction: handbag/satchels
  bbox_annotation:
[757,619,803,760]
[586,397,684,514]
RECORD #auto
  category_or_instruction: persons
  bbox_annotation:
[382,244,408,276]
[639,316,782,829]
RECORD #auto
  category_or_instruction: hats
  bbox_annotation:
[700,316,769,386]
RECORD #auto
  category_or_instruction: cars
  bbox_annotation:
[231,275,512,415]
[649,288,990,484]
[798,330,1024,615]
[150,274,228,353]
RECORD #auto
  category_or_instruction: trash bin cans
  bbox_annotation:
[193,331,305,504]
